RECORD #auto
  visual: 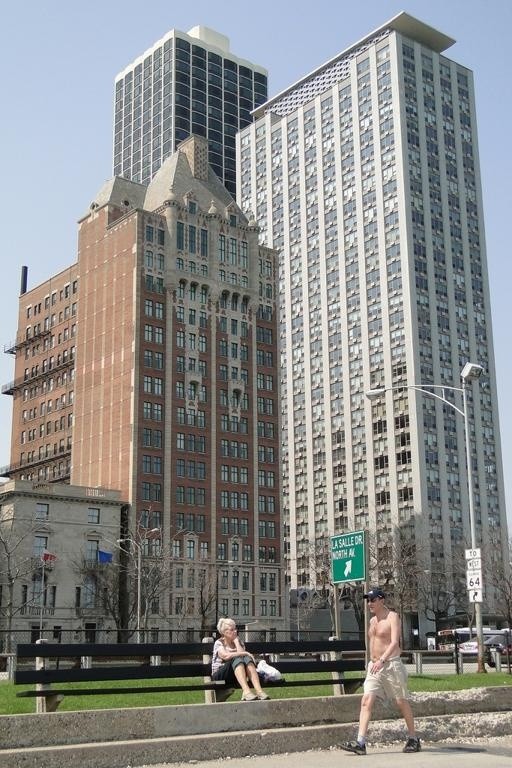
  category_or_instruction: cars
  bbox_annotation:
[456,630,512,668]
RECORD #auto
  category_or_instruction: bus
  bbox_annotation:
[436,626,512,654]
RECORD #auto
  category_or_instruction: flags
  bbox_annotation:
[42,551,56,561]
[97,551,113,564]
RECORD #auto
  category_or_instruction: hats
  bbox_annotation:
[362,589,385,600]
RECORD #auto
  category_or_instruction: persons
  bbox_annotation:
[210,616,269,701]
[335,586,423,755]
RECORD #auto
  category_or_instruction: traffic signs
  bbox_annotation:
[329,529,367,585]
[464,549,481,559]
[465,569,483,590]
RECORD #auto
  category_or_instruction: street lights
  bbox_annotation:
[364,359,490,675]
[116,526,160,644]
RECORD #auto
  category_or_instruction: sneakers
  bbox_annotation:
[256,691,269,700]
[241,690,258,701]
[337,739,367,755]
[403,736,421,753]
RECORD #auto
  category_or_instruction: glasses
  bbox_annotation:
[366,597,376,604]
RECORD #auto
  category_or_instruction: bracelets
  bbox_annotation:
[379,658,385,664]
